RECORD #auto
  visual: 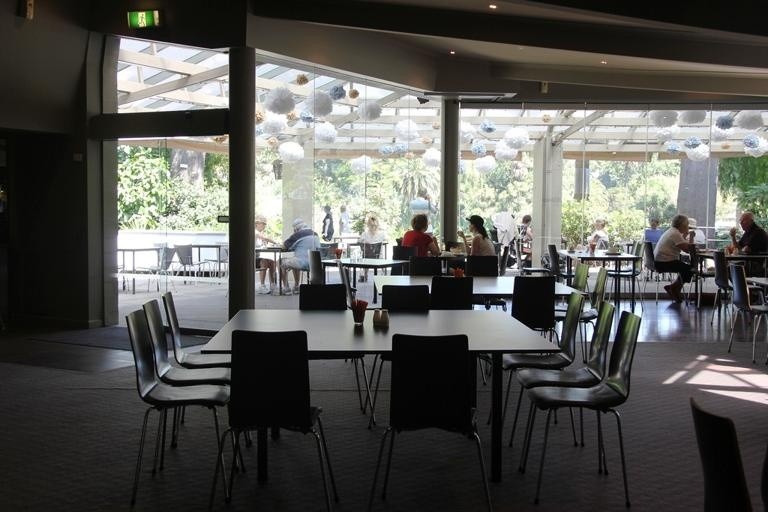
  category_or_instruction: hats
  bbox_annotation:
[466,215,484,226]
[688,217,696,229]
[256,215,267,225]
[293,219,305,229]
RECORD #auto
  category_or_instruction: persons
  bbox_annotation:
[683,218,706,243]
[456,215,497,260]
[400,212,441,258]
[410,189,437,237]
[322,205,334,243]
[336,205,349,236]
[726,211,768,285]
[645,218,663,242]
[358,217,386,283]
[586,218,608,248]
[652,215,697,304]
[516,214,534,275]
[279,218,319,296]
[252,213,280,295]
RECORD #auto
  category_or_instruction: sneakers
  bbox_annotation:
[258,285,271,294]
[281,288,293,296]
[293,287,300,294]
[270,286,281,296]
[664,285,683,303]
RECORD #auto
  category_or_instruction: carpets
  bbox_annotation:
[25,327,211,353]
[0,344,768,512]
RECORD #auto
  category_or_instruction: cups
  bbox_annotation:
[350,300,368,326]
[335,250,342,259]
[590,243,595,254]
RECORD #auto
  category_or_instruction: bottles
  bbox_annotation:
[381,309,388,327]
[372,309,380,326]
